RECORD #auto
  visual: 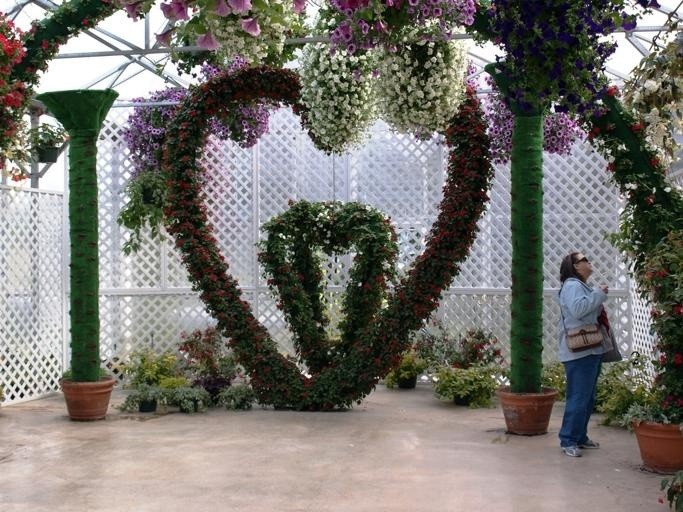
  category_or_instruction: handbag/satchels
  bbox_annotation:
[565,322,604,352]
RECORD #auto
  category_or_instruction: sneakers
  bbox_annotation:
[560,445,582,457]
[577,437,599,449]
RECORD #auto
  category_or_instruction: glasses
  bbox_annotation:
[575,256,588,264]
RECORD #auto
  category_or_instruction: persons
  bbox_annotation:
[557,251,624,457]
